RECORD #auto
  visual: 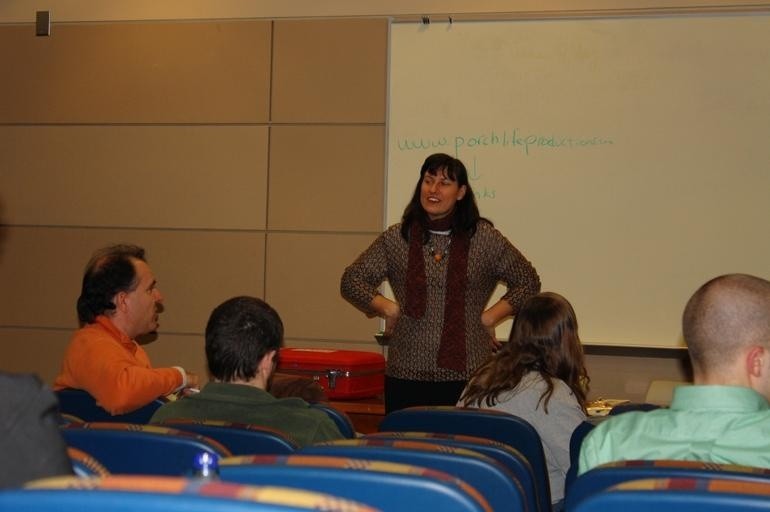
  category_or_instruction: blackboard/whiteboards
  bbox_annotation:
[373,4,770,358]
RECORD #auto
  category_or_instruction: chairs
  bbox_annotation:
[0,388,769,512]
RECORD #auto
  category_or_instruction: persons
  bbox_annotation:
[52,242,200,420]
[339,152,542,422]
[457,290,591,511]
[1,369,77,498]
[574,272,770,480]
[142,294,348,445]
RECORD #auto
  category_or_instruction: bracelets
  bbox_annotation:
[170,365,188,394]
